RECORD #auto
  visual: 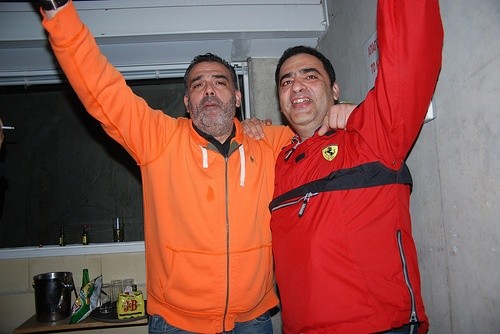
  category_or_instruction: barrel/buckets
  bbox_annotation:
[32,272,73,321]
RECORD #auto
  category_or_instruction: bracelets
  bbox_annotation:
[31,0,69,10]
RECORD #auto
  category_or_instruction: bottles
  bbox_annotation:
[59,225,65,246]
[82,225,89,245]
[80,269,89,292]
[132,285,137,292]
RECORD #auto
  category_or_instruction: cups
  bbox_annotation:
[112,280,122,301]
[122,279,134,288]
[112,218,124,242]
[100,287,112,313]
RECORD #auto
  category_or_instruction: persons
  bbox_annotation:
[238,0,445,333]
[31,0,358,334]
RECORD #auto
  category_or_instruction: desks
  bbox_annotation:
[14,299,149,334]
